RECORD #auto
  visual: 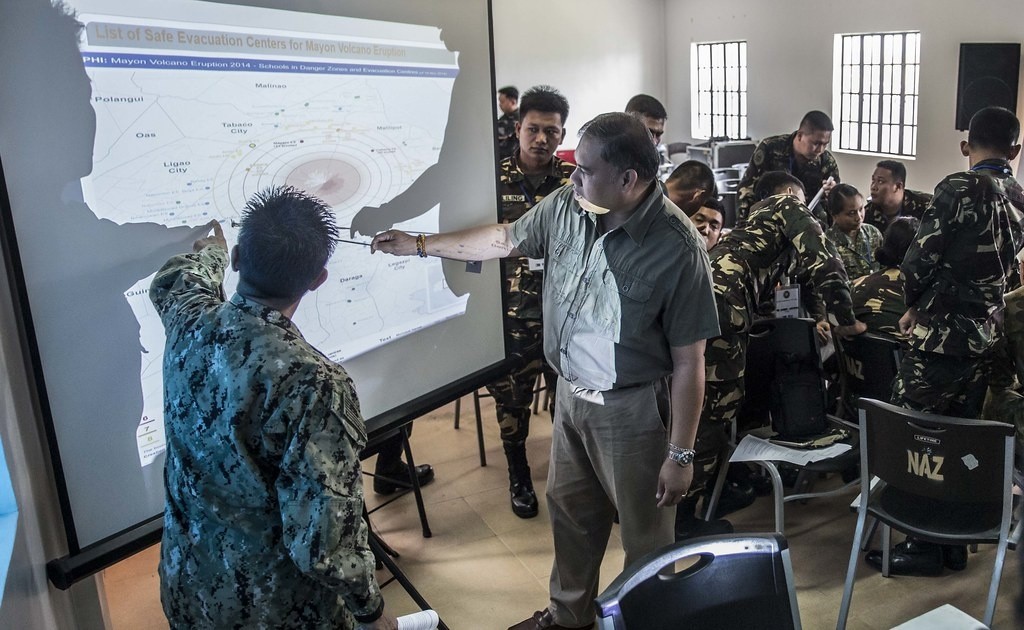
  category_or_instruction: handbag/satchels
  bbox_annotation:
[772,365,825,439]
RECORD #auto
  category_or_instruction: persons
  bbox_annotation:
[660,107,1024,578]
[487,86,578,519]
[147,185,398,630]
[370,112,721,630]
[497,86,523,158]
[626,94,667,146]
[371,421,434,495]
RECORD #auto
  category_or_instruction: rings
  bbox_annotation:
[682,492,687,497]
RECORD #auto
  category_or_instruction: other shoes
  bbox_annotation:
[750,472,772,489]
[780,465,799,486]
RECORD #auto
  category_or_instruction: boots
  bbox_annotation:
[700,476,757,519]
[674,495,734,542]
[503,443,539,517]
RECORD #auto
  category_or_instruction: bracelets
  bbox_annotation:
[668,442,696,453]
[416,234,427,258]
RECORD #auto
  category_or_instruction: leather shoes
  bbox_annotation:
[507,608,594,630]
[939,544,968,571]
[374,462,435,495]
[865,536,945,576]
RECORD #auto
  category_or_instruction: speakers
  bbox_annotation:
[761,318,826,440]
[955,42,1021,132]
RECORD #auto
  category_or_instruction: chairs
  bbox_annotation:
[596,306,1024,630]
[655,136,758,229]
[353,420,433,571]
[451,371,550,467]
[368,530,449,630]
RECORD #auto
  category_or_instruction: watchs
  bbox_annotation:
[668,451,695,466]
